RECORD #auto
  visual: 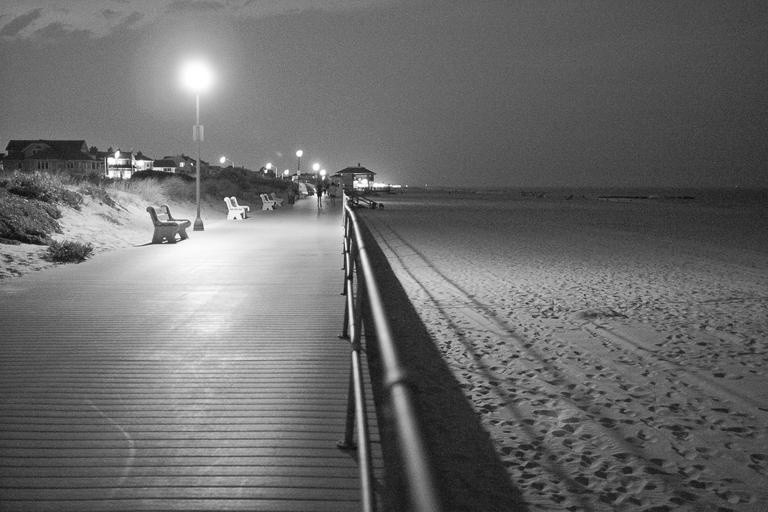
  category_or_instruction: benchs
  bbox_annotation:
[260,192,295,211]
[224,196,250,220]
[146,203,190,243]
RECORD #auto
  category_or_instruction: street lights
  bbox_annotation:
[280,169,288,179]
[185,67,206,231]
[218,156,234,168]
[295,149,301,200]
[313,163,319,184]
[266,163,277,178]
